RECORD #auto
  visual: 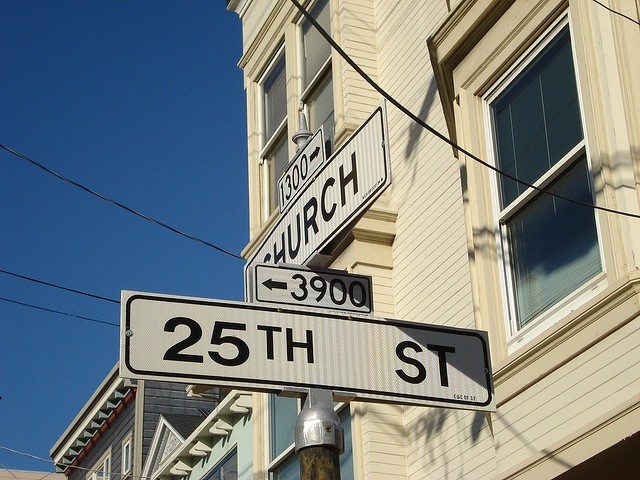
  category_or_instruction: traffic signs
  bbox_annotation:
[119,289,497,413]
[276,124,326,214]
[253,262,373,317]
[244,99,392,303]
